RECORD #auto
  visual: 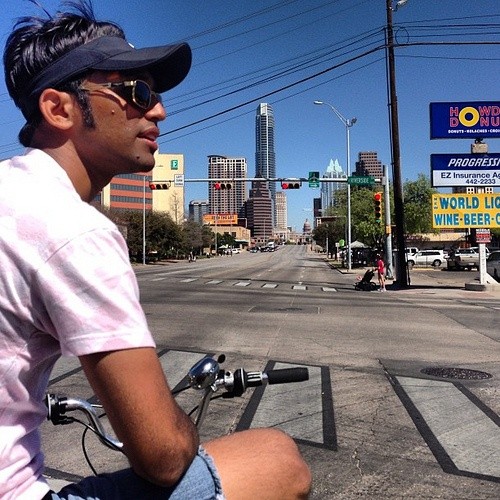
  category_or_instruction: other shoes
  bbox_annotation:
[381,289,387,292]
[377,287,382,291]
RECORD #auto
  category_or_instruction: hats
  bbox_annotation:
[14,31,192,106]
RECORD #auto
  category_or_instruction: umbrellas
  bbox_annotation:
[344,240,372,248]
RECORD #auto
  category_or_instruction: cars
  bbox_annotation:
[486,250,500,282]
[250,242,280,253]
[224,249,241,255]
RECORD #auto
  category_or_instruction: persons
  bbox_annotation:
[352,247,364,265]
[0,0,313,500]
[375,255,387,292]
[340,251,349,269]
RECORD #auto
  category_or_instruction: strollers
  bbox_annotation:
[352,268,377,292]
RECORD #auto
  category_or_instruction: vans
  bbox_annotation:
[404,244,492,268]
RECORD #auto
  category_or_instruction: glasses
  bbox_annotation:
[65,78,164,112]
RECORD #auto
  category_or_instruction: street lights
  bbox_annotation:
[313,100,357,273]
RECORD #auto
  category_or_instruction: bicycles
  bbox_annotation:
[44,349,310,475]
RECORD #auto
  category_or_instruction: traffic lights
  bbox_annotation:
[214,183,232,190]
[282,181,301,189]
[149,183,169,189]
[374,193,382,224]
